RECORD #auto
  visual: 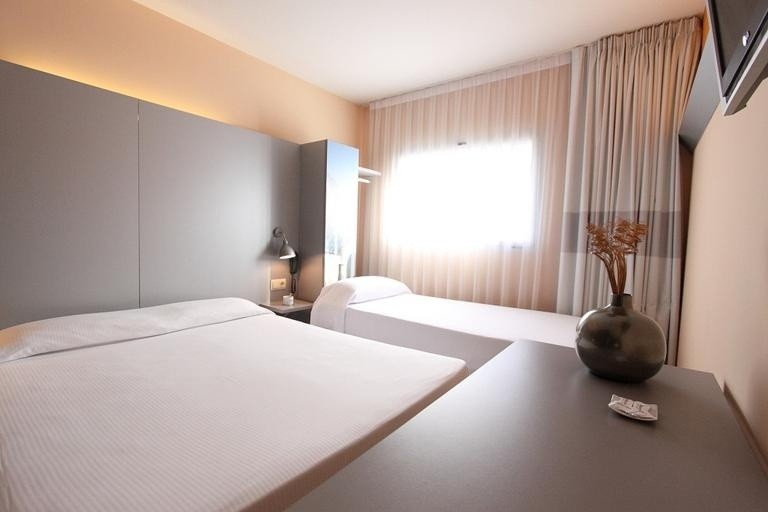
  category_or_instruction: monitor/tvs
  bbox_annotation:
[707,0,768,117]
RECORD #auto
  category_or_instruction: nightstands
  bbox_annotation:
[258,298,313,324]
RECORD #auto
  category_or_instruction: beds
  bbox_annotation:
[310,275,581,372]
[0,297,469,511]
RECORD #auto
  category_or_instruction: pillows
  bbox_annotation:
[309,275,412,331]
[1,297,276,363]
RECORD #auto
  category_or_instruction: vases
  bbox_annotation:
[575,293,668,385]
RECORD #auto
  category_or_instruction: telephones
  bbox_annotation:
[289,251,299,294]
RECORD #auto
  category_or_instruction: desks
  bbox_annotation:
[283,337,768,512]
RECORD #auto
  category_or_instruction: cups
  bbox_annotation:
[283,295,294,306]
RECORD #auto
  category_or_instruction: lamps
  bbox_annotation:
[274,227,297,260]
